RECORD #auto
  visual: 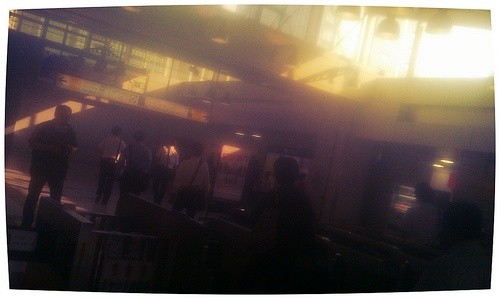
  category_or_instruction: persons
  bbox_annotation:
[398,181,445,239]
[167,142,210,219]
[93,126,127,206]
[139,157,402,292]
[412,200,490,288]
[21,105,77,229]
[111,130,153,215]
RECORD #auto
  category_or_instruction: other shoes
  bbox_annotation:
[95,196,100,201]
[102,200,107,204]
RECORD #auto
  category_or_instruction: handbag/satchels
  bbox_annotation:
[189,190,205,211]
